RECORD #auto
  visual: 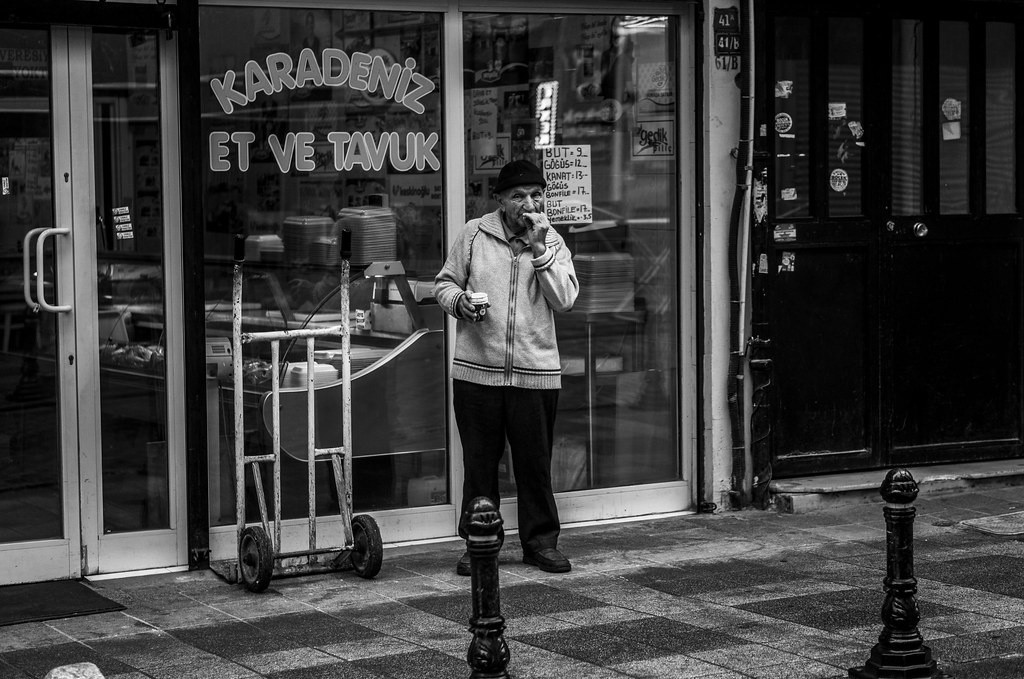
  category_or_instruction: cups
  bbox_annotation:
[470,293,488,322]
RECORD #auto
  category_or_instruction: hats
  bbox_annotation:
[494,159,547,194]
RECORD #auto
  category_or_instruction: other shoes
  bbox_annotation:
[457,552,471,576]
[522,547,571,573]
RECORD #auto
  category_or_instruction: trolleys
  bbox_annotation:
[214,230,384,588]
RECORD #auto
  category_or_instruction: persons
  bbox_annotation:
[436,158,581,575]
